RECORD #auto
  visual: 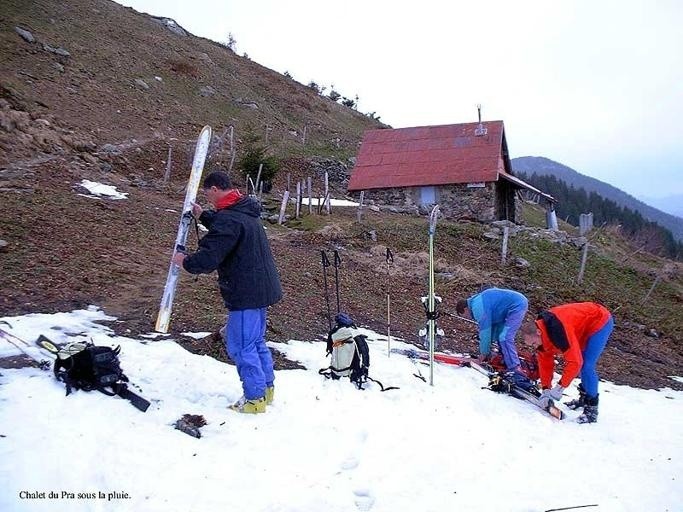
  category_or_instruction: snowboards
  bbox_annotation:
[156,123,212,332]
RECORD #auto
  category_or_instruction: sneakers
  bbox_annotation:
[226,394,266,413]
[265,385,274,405]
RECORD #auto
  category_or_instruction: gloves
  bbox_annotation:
[550,383,565,401]
[539,389,553,409]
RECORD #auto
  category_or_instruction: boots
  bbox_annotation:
[573,394,599,424]
[563,383,587,410]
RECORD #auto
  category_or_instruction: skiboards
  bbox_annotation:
[424,204,438,385]
[1,322,199,437]
[390,347,492,367]
[468,359,565,419]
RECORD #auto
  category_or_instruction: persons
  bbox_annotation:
[455,287,529,370]
[171,172,286,415]
[518,302,614,424]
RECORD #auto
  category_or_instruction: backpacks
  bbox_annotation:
[326,322,370,383]
[52,341,129,398]
[488,349,540,381]
